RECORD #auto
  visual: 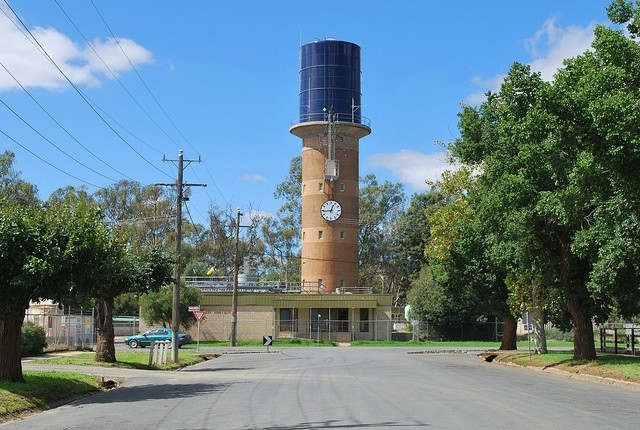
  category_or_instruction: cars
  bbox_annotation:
[125,327,191,349]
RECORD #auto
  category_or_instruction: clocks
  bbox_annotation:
[319,199,343,222]
